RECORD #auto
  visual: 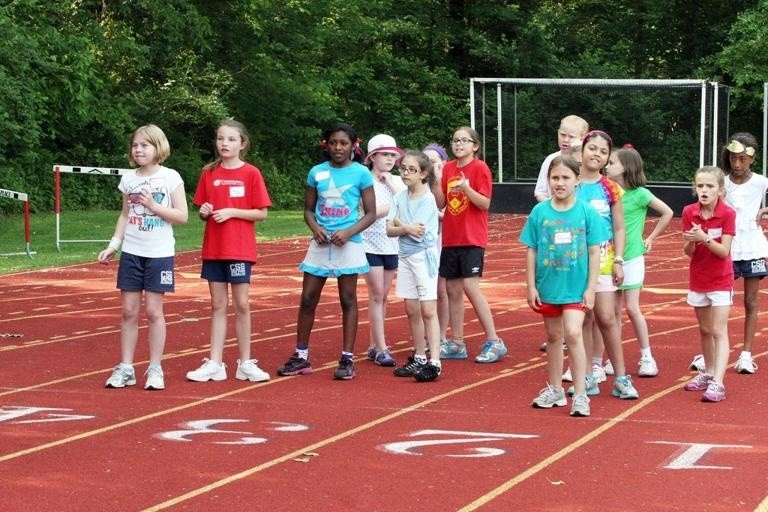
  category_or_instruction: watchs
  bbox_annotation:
[702,235,711,246]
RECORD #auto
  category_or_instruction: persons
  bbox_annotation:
[518,155,604,417]
[680,164,736,403]
[276,124,379,382]
[357,127,508,382]
[184,119,273,384]
[98,122,190,391]
[535,114,674,399]
[687,131,768,374]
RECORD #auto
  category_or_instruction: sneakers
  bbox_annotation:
[684,374,713,391]
[415,364,441,381]
[439,340,448,354]
[448,341,465,353]
[567,377,600,395]
[475,338,507,362]
[639,356,658,376]
[395,357,429,376]
[374,347,396,366]
[688,355,705,370]
[541,342,568,351]
[334,357,356,379]
[368,348,391,360]
[277,352,312,376]
[592,364,606,383]
[144,365,165,390]
[734,355,758,374]
[603,359,615,374]
[106,365,136,387]
[702,380,725,401]
[570,394,590,416]
[186,358,227,382]
[563,367,574,381]
[533,381,567,408]
[236,359,270,382]
[612,375,640,398]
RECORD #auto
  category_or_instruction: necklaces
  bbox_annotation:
[727,169,750,192]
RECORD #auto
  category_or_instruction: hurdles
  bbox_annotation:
[52,165,133,254]
[0,188,37,259]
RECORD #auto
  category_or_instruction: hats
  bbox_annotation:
[364,134,404,166]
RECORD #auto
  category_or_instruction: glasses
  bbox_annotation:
[451,137,475,145]
[397,166,422,174]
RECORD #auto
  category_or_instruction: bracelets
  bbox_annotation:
[109,235,122,253]
